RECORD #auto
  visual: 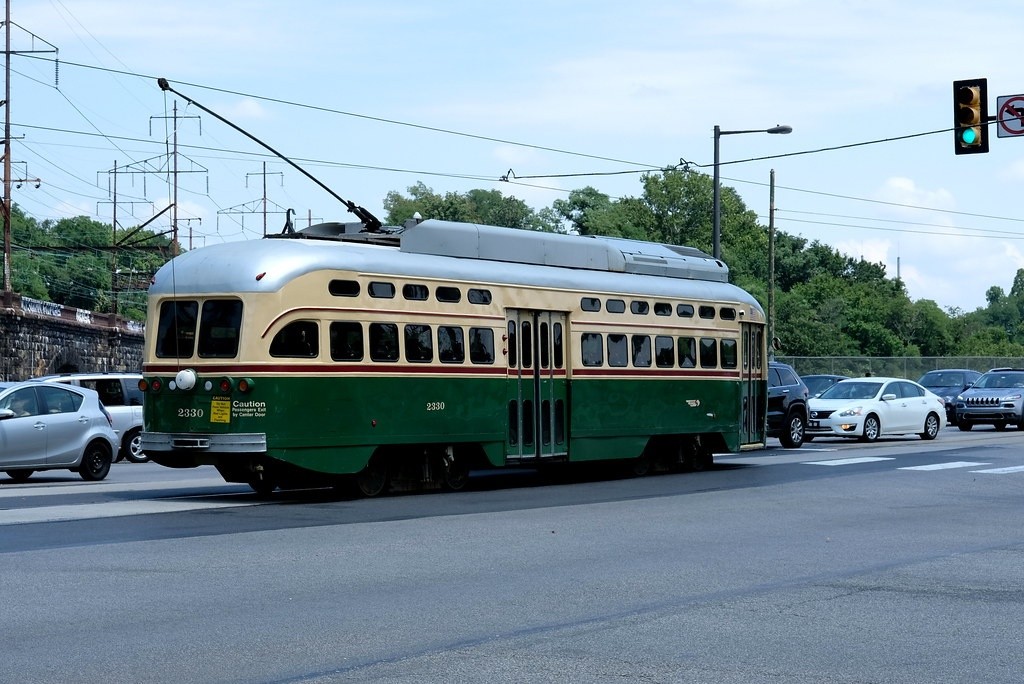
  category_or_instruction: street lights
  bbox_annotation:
[711,124,794,259]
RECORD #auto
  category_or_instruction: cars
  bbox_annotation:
[954,367,1024,431]
[915,369,984,428]
[805,377,947,444]
[0,383,122,482]
[799,374,856,400]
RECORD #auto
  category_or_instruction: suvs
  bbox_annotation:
[767,362,811,448]
[22,371,150,463]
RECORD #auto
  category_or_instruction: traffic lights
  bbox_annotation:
[953,77,991,156]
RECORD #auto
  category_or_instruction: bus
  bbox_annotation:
[134,77,771,499]
